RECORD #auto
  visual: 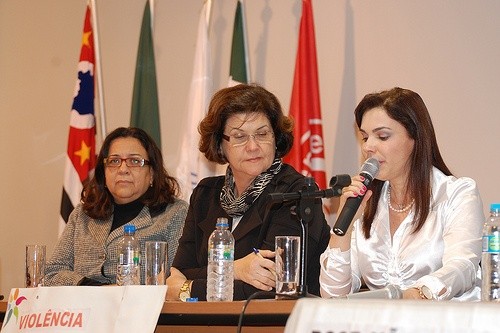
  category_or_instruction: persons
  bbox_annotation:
[41,128,189,286]
[319,86,487,300]
[154,85,330,305]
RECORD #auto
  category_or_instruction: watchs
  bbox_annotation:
[178,279,193,302]
[412,285,433,300]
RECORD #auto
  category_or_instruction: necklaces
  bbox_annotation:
[387,182,415,213]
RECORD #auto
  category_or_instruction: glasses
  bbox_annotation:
[102,157,150,167]
[222,130,274,147]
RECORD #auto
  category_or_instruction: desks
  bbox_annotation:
[0,301,296,333]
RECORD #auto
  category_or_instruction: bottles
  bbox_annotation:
[115,225,141,286]
[481,203,500,305]
[207,217,235,302]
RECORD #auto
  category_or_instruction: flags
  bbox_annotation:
[127,0,329,215]
[59,0,98,246]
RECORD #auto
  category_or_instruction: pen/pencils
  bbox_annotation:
[253,247,278,277]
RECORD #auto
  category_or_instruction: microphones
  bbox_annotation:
[333,158,380,236]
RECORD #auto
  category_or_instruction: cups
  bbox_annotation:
[25,245,46,288]
[145,241,169,285]
[275,236,300,300]
